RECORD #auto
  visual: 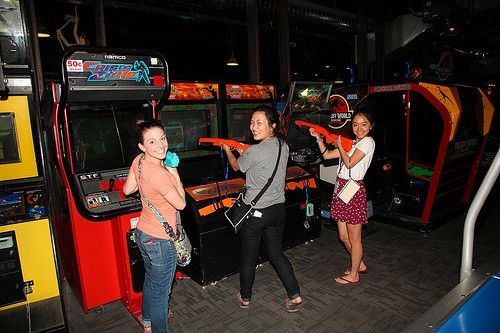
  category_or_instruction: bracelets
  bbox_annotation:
[316,137,323,143]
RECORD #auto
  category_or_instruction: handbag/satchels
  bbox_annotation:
[222,193,256,233]
[173,226,192,267]
[337,178,361,205]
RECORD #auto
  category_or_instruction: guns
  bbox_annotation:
[196,135,251,155]
[163,151,180,169]
[294,119,353,153]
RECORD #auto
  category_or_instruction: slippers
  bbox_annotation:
[236,292,249,309]
[344,267,368,276]
[333,274,362,285]
[284,298,300,312]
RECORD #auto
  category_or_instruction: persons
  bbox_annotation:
[220,105,302,313]
[309,108,375,285]
[123,120,187,333]
